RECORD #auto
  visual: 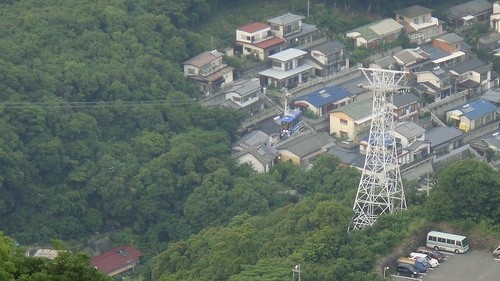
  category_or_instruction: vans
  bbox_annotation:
[396,255,432,278]
[410,252,439,268]
[493,246,500,259]
[418,247,443,263]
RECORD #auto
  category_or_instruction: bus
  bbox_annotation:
[280,109,303,138]
[427,230,469,254]
